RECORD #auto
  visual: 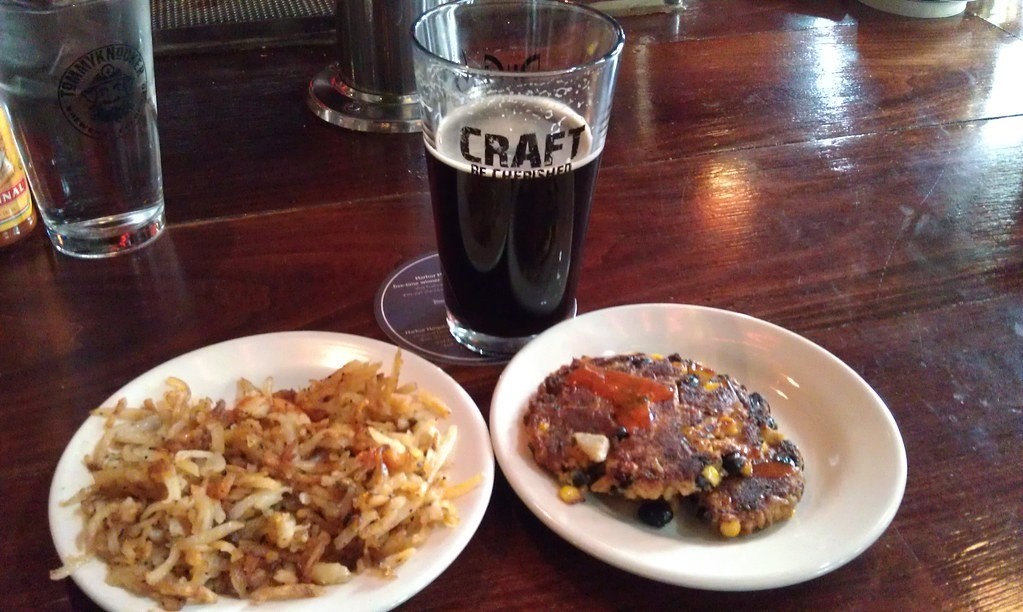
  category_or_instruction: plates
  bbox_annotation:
[488,303,908,591]
[47,330,496,612]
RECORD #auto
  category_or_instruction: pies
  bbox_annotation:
[522,352,777,501]
[695,427,806,536]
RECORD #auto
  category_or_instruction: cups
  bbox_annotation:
[332,0,426,105]
[409,0,626,359]
[0,0,167,260]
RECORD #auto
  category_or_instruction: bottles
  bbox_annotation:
[0,108,38,249]
